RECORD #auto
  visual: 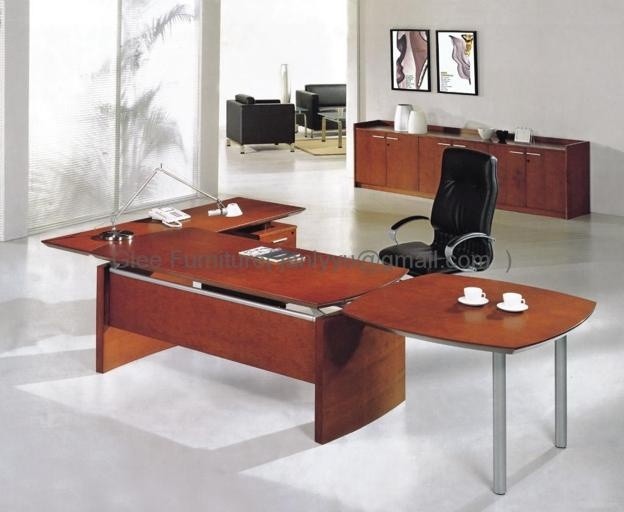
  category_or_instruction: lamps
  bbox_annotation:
[98,161,243,241]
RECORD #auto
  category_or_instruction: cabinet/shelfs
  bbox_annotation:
[352,119,590,221]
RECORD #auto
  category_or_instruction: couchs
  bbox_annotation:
[294,83,346,138]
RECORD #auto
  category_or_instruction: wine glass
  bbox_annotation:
[496,130,509,144]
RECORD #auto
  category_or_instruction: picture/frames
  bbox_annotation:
[389,28,478,96]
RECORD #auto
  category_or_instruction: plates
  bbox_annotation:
[458,297,490,306]
[497,302,530,313]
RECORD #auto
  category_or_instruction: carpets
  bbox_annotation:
[293,138,345,157]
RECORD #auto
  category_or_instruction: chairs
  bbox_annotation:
[378,146,512,279]
[226,84,294,153]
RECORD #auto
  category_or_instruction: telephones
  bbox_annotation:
[149,206,191,223]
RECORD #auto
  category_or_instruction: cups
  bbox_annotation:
[502,291,526,307]
[463,286,487,302]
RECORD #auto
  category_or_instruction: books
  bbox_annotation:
[238,245,281,257]
[257,248,302,264]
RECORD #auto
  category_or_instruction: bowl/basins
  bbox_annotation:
[478,125,497,141]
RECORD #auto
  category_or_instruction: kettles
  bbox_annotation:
[394,104,413,132]
